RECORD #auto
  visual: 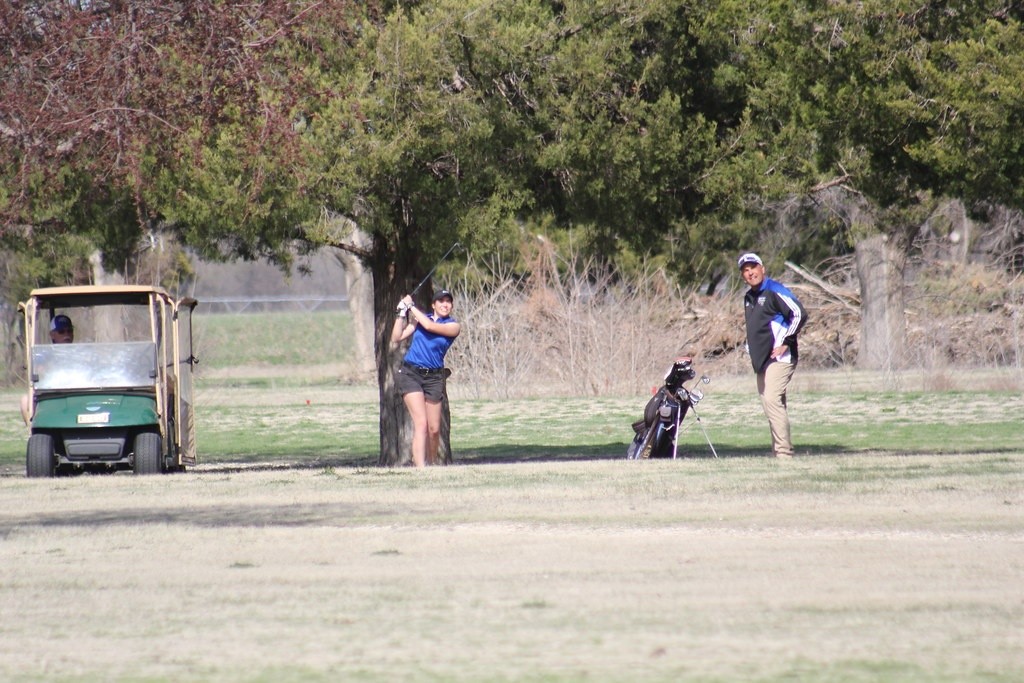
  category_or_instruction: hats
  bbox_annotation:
[738,253,762,270]
[49,315,75,331]
[432,289,453,302]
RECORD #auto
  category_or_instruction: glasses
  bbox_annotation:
[55,327,74,334]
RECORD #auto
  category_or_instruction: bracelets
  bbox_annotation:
[406,302,415,310]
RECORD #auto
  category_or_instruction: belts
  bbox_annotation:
[400,361,445,376]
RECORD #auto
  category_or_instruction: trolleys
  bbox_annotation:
[625,357,720,461]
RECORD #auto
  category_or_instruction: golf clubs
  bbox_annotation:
[396,243,462,314]
[665,359,710,404]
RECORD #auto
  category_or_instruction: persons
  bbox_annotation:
[738,252,809,458]
[20,314,74,436]
[387,290,462,469]
